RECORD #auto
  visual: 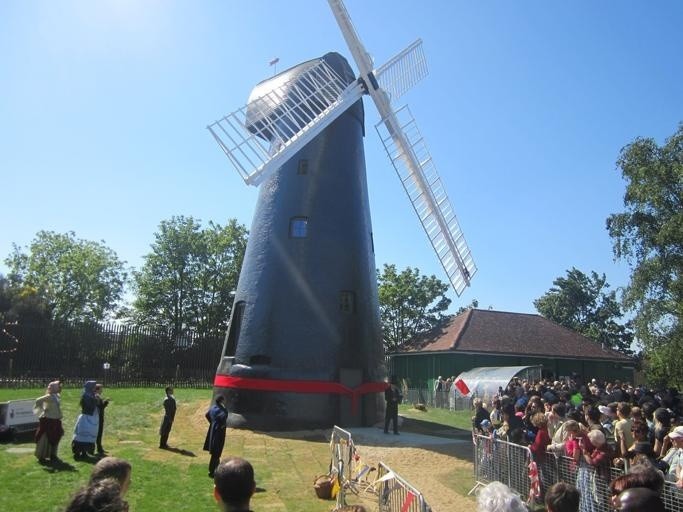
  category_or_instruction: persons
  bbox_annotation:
[384,381,405,435]
[433,377,682,511]
[72,381,98,458]
[87,458,130,498]
[62,477,130,512]
[33,381,62,465]
[202,394,227,476]
[95,384,108,455]
[158,389,175,449]
[213,458,257,512]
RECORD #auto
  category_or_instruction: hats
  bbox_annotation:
[389,374,398,384]
[669,426,683,439]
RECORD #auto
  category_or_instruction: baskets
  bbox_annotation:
[313,475,334,499]
